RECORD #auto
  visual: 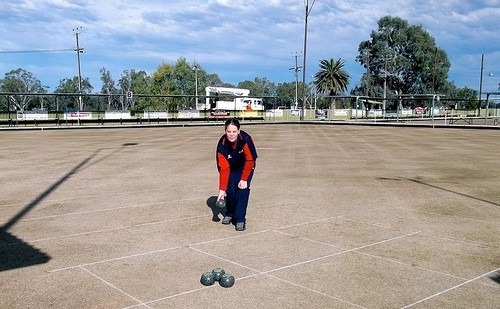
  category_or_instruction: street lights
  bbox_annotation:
[190,63,200,116]
[479,53,493,107]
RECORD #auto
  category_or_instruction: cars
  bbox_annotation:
[411,104,432,116]
[206,107,235,119]
[266,105,291,115]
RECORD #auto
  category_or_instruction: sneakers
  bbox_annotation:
[222,216,232,224]
[236,222,244,231]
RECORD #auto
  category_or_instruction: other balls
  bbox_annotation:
[216,199,226,208]
[200,266,235,289]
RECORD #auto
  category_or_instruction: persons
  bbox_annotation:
[216,118,258,231]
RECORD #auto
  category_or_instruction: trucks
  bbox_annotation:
[215,97,266,120]
